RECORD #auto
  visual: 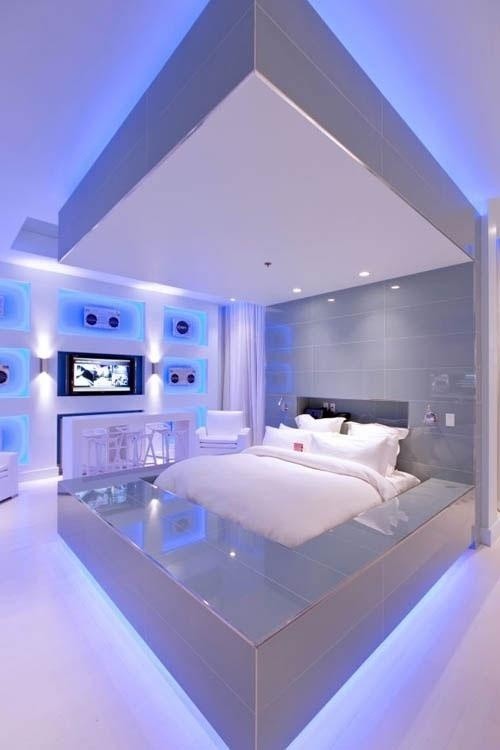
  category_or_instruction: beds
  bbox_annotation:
[153,422,421,547]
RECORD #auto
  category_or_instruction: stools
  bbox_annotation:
[82,423,168,478]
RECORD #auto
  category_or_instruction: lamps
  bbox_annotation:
[278,392,288,412]
[424,403,436,424]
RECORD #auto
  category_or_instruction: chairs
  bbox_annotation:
[195,410,251,457]
[0,452,19,501]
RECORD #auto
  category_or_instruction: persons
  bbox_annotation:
[75,365,99,382]
[107,366,112,381]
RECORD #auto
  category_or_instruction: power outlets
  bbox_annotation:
[324,403,336,412]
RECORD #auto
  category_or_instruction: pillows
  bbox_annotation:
[263,414,410,476]
